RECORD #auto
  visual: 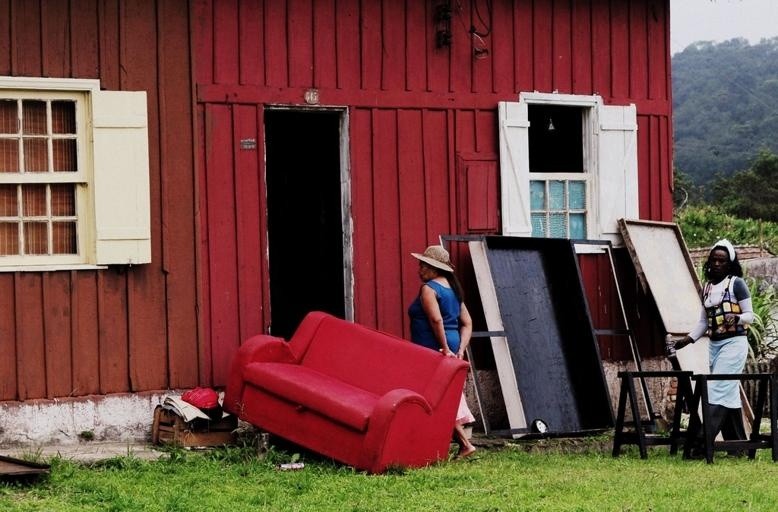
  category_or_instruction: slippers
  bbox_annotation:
[451,454,482,465]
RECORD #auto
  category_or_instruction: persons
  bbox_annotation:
[408,246,476,460]
[671,238,755,460]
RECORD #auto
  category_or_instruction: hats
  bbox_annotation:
[410,245,456,272]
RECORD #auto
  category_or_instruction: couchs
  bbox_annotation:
[221,311,469,474]
